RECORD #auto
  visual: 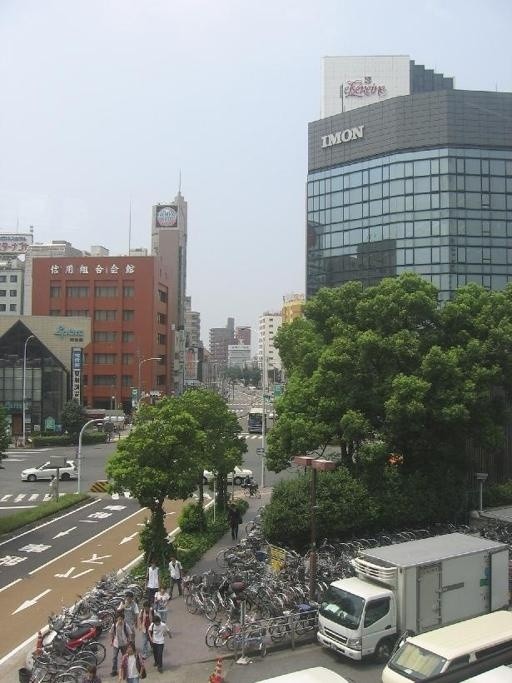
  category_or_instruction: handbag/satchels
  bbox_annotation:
[139,665,146,678]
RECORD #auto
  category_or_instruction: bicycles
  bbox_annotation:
[183,506,512,657]
[244,481,261,499]
[28,566,144,683]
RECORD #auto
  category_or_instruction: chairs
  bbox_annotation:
[365,605,376,622]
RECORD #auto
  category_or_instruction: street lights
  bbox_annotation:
[242,326,267,487]
[22,335,37,447]
[138,357,163,401]
[77,418,108,493]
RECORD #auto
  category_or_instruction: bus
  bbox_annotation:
[248,408,266,433]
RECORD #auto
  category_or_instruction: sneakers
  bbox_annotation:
[153,662,156,667]
[110,671,117,674]
[159,666,162,673]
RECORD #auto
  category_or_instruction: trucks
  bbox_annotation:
[316,531,509,662]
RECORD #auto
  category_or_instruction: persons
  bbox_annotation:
[108,612,133,677]
[47,474,58,497]
[144,558,161,609]
[243,474,259,497]
[167,553,184,600]
[137,599,160,659]
[117,640,144,683]
[153,583,172,624]
[147,614,172,674]
[116,591,141,635]
[226,503,240,541]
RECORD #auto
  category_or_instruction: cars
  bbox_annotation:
[202,466,254,486]
[21,460,77,481]
[268,409,277,419]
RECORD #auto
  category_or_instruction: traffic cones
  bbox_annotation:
[209,655,225,683]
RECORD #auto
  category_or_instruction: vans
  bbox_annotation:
[381,610,512,683]
